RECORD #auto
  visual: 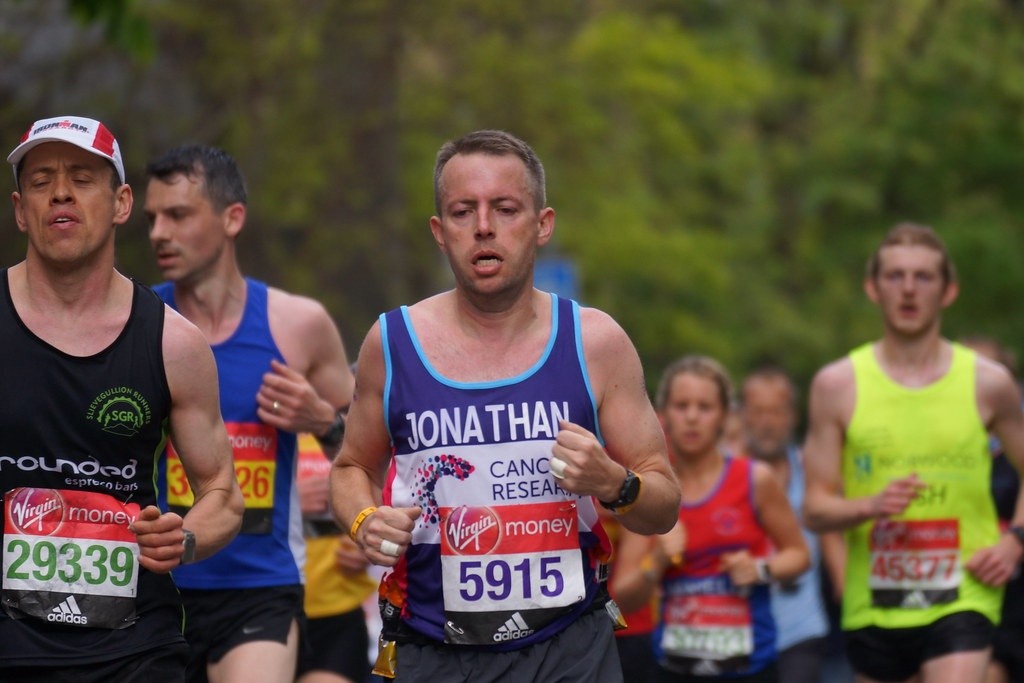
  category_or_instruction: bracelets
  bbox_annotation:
[1009,526,1024,543]
[757,559,771,583]
[595,472,643,515]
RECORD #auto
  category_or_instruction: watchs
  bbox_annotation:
[318,413,344,444]
[599,469,641,511]
[180,529,195,565]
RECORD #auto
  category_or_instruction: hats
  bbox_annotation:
[7,115,125,191]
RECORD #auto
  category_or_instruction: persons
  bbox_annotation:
[292,128,680,682]
[0,116,247,683]
[126,139,359,683]
[600,222,1024,683]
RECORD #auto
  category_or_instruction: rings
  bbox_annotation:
[549,458,566,479]
[351,506,377,544]
[380,540,398,557]
[273,403,279,414]
